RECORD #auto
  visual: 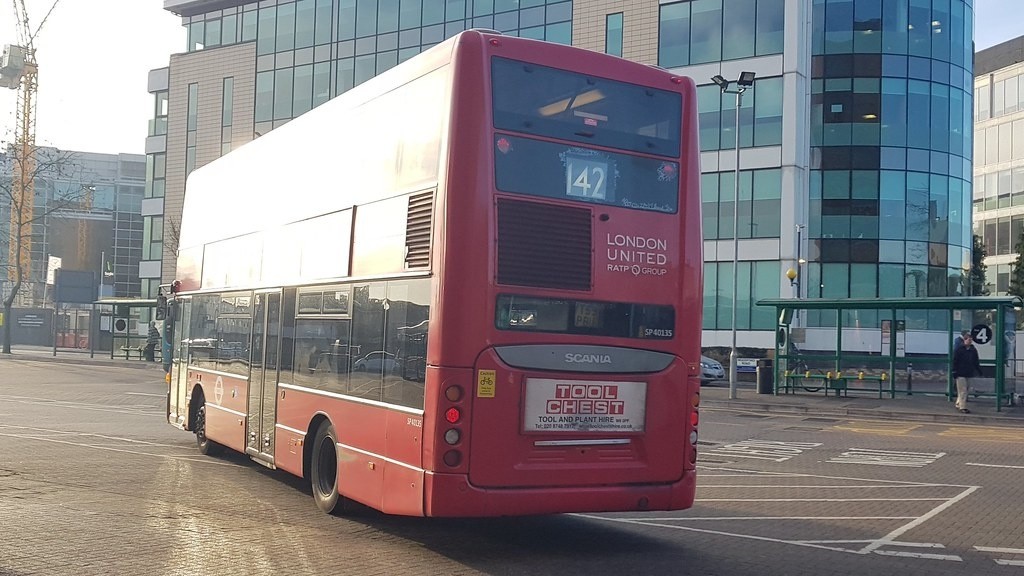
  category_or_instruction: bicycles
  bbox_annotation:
[788,354,825,392]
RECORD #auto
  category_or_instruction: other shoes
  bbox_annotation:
[960,409,969,413]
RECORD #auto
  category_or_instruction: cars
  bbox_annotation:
[701,355,725,385]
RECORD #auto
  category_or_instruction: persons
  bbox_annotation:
[954,330,971,349]
[145,321,159,361]
[953,335,980,413]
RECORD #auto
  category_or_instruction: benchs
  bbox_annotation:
[784,371,834,396]
[836,371,889,399]
[119,345,143,361]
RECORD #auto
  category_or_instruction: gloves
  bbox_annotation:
[952,372,957,379]
[977,367,983,373]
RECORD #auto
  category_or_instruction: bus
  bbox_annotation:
[155,30,704,519]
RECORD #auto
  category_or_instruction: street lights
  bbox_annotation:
[710,71,756,400]
